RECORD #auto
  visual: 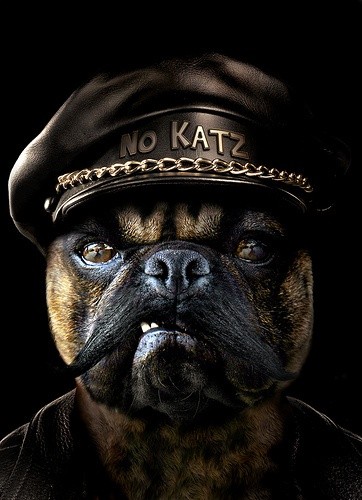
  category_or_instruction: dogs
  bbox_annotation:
[43,182,316,500]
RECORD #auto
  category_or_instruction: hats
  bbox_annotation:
[8,49,358,254]
